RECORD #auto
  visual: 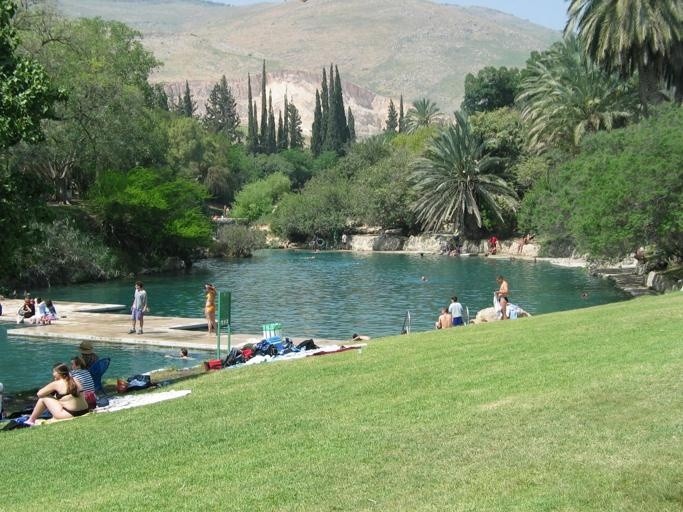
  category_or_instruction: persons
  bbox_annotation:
[204,284,218,337]
[13,287,59,325]
[341,231,347,250]
[487,233,498,255]
[448,296,464,327]
[127,280,148,334]
[435,307,452,330]
[438,244,458,256]
[180,348,188,359]
[421,276,428,281]
[23,341,103,427]
[352,333,371,340]
[494,275,509,320]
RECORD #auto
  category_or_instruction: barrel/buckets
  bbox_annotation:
[263,323,284,343]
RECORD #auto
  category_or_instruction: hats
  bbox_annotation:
[78,340,94,355]
